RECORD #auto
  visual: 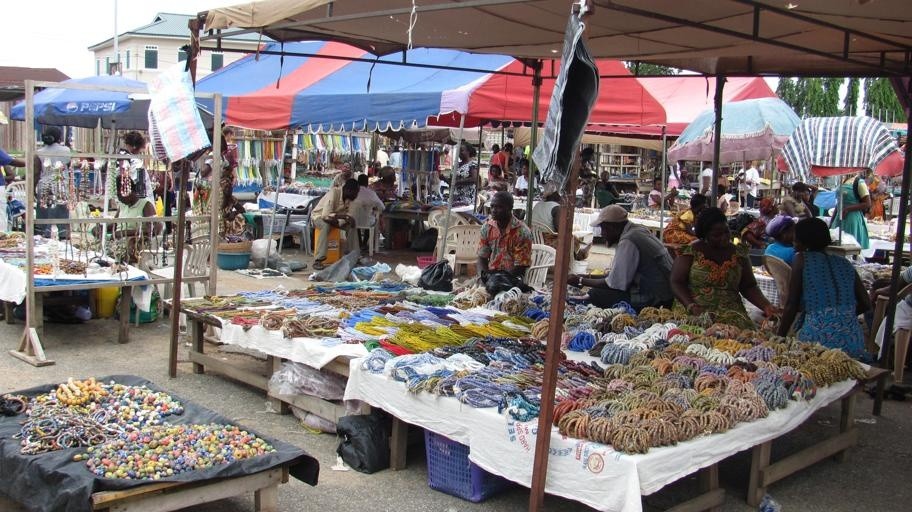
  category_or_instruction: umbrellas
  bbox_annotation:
[9,72,227,254]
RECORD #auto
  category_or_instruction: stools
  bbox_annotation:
[314,226,347,264]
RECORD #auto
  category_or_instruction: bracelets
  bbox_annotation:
[12,394,122,454]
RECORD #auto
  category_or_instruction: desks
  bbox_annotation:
[0,239,149,348]
[247,212,306,232]
[572,210,859,255]
[158,290,888,511]
[0,371,293,512]
[384,205,477,249]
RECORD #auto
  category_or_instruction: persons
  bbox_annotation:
[90,174,163,266]
[221,175,247,237]
[78,132,154,208]
[165,176,177,232]
[223,127,240,187]
[192,128,227,240]
[306,139,561,283]
[32,126,72,241]
[0,148,28,237]
[567,149,911,399]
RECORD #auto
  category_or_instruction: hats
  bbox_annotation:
[760,197,775,215]
[766,215,793,238]
[589,205,628,227]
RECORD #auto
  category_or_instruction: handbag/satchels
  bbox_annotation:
[417,260,453,291]
[480,269,533,295]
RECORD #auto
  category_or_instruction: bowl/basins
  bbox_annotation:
[216,252,252,270]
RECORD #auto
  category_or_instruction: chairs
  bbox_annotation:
[151,235,225,315]
[428,210,558,291]
[762,254,792,307]
[348,201,382,257]
[278,195,321,255]
[140,221,166,263]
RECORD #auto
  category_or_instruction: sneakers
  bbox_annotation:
[312,261,325,270]
